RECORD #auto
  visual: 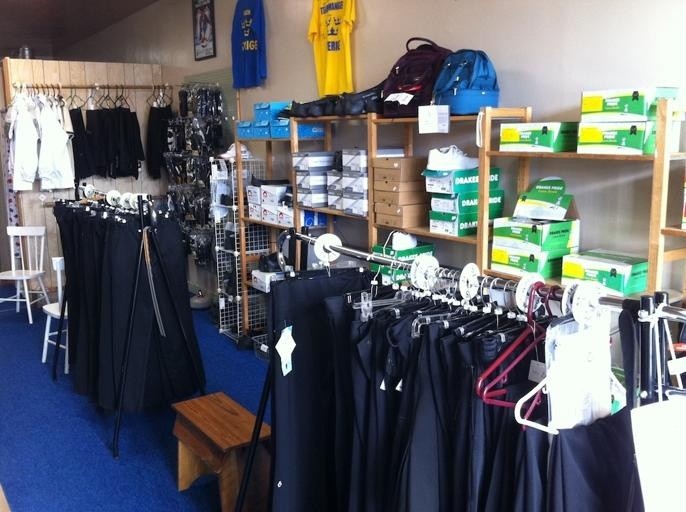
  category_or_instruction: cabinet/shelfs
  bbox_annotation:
[205,158,275,343]
[235,92,686,337]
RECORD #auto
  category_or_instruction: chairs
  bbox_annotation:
[2,224,49,326]
[39,251,76,375]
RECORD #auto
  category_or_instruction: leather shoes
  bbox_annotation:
[275,77,384,119]
[251,173,290,187]
[221,193,271,336]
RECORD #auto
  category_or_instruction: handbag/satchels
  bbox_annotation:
[258,253,289,272]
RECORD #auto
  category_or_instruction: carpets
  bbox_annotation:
[0,282,285,511]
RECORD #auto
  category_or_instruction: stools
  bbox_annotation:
[170,390,270,511]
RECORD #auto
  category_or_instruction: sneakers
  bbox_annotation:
[390,231,419,251]
[426,145,480,171]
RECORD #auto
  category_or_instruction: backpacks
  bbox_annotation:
[381,44,453,118]
[431,48,500,115]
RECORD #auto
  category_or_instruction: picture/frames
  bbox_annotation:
[192,1,216,60]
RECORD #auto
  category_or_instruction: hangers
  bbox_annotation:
[12,75,173,113]
[56,177,178,231]
